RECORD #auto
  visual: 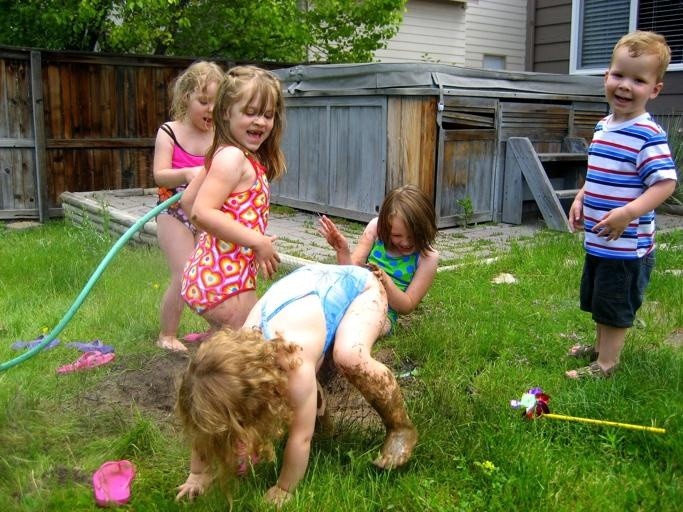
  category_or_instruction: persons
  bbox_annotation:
[151,60,227,353]
[316,183,438,339]
[564,30,678,382]
[170,264,418,511]
[178,64,288,475]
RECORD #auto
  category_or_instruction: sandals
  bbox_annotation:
[565,345,614,379]
